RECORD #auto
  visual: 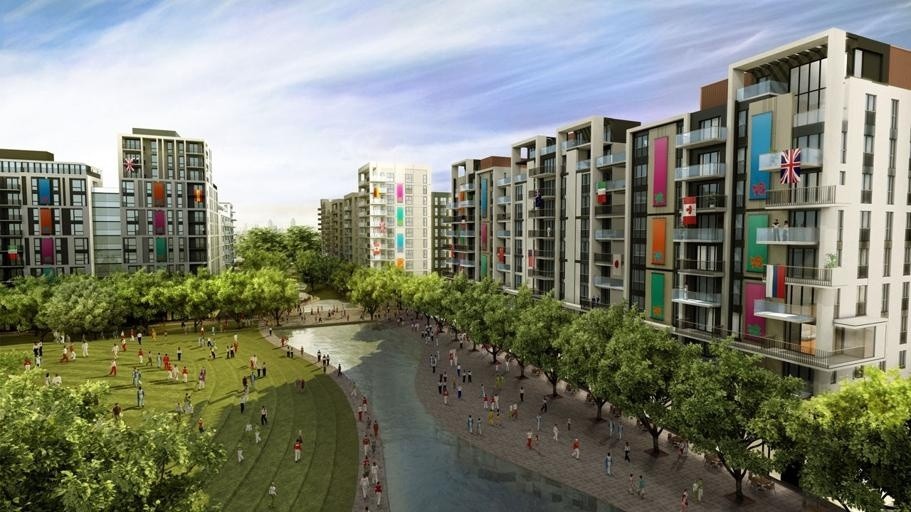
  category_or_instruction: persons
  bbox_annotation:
[591,296,596,308]
[782,220,788,241]
[596,296,601,306]
[387,300,705,511]
[771,219,780,241]
[22,300,383,511]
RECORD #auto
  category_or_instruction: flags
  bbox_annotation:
[373,241,379,254]
[123,159,135,171]
[613,252,621,271]
[780,148,801,184]
[8,245,18,261]
[683,197,697,225]
[597,181,606,203]
[765,265,785,299]
[380,221,385,233]
